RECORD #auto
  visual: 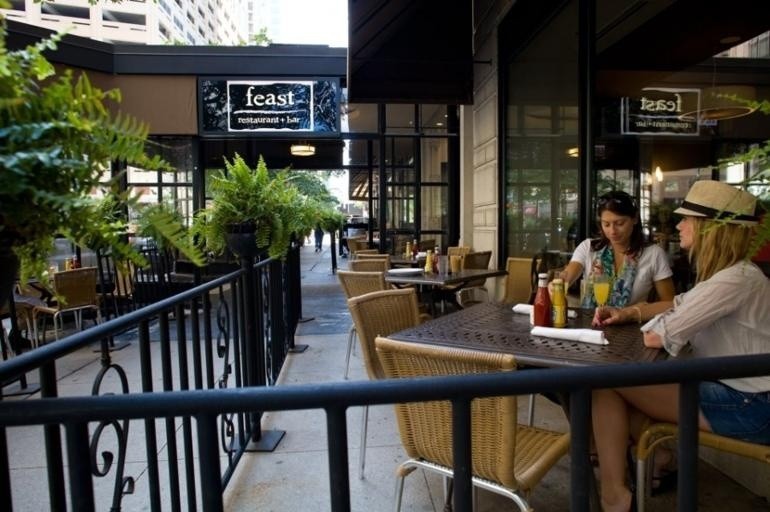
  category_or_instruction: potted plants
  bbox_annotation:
[0,16,171,290]
[183,152,347,264]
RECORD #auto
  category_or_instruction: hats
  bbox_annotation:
[673,178,758,227]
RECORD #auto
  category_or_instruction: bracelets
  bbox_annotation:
[632,305,642,324]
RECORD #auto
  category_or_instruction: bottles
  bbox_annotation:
[553,280,568,327]
[433,247,441,273]
[413,238,418,260]
[535,271,552,326]
[424,250,434,274]
[405,241,412,261]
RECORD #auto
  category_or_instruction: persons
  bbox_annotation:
[557,189,674,314]
[590,179,770,512]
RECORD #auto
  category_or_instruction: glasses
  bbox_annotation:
[597,197,621,206]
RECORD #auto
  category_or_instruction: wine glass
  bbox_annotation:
[593,280,611,332]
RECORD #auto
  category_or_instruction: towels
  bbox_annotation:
[531,327,608,345]
[514,303,577,316]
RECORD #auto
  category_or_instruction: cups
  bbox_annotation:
[438,255,449,280]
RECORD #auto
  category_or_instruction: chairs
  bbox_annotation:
[33,266,99,347]
[340,274,387,378]
[442,254,492,312]
[526,254,562,306]
[358,255,388,268]
[347,259,385,276]
[448,246,474,261]
[376,336,572,511]
[418,240,435,253]
[498,254,541,306]
[348,238,362,255]
[356,249,377,258]
[636,419,769,512]
[345,288,421,481]
